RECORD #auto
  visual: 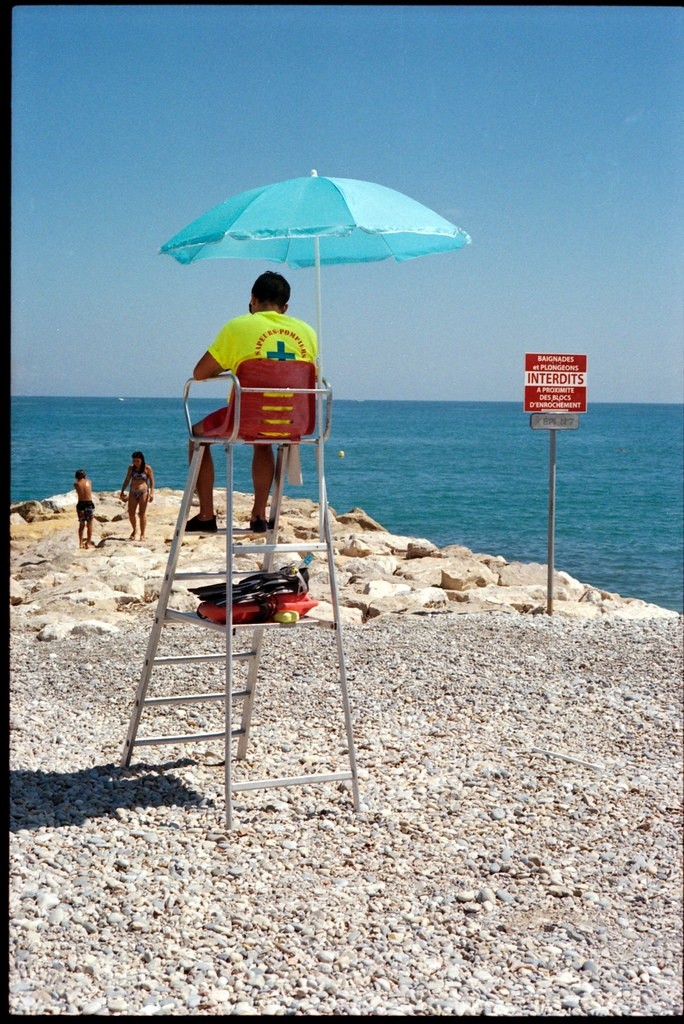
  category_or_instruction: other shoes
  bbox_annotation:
[250,516,267,534]
[185,514,218,533]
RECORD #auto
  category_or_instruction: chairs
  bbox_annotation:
[203,358,316,440]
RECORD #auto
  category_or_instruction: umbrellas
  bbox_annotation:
[158,172,472,540]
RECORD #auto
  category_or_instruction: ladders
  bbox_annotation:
[119,444,292,769]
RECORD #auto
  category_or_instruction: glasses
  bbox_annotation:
[249,300,252,313]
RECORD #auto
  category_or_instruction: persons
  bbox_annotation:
[120,450,154,541]
[184,271,320,533]
[74,469,95,550]
